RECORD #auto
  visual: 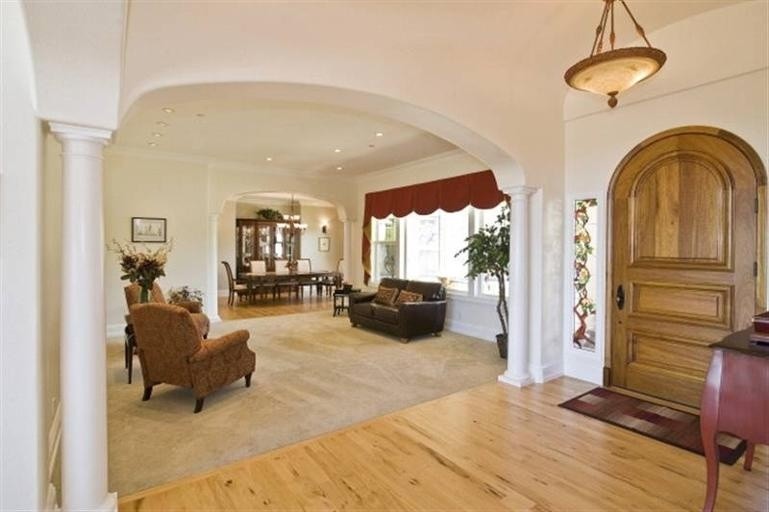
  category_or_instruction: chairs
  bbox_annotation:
[124,281,210,368]
[130,303,256,414]
[221,261,343,305]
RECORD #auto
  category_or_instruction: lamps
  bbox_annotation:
[276,193,308,236]
[564,0,667,108]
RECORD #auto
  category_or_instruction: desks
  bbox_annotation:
[699,325,769,512]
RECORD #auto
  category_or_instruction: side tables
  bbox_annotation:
[332,293,352,316]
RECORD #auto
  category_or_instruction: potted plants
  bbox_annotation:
[453,209,509,358]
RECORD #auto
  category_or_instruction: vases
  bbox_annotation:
[140,292,148,303]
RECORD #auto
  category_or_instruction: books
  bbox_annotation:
[335,283,360,293]
[748,311,769,346]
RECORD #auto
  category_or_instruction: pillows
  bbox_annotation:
[406,280,443,301]
[373,287,399,306]
[395,290,424,308]
[380,278,410,292]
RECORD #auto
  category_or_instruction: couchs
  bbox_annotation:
[348,278,448,343]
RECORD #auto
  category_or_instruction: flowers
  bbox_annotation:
[105,235,176,301]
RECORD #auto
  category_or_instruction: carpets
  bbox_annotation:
[105,308,508,497]
[557,385,747,465]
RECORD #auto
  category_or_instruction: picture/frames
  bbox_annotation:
[318,236,331,253]
[131,217,166,243]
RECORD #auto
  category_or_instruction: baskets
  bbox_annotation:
[168,293,203,313]
